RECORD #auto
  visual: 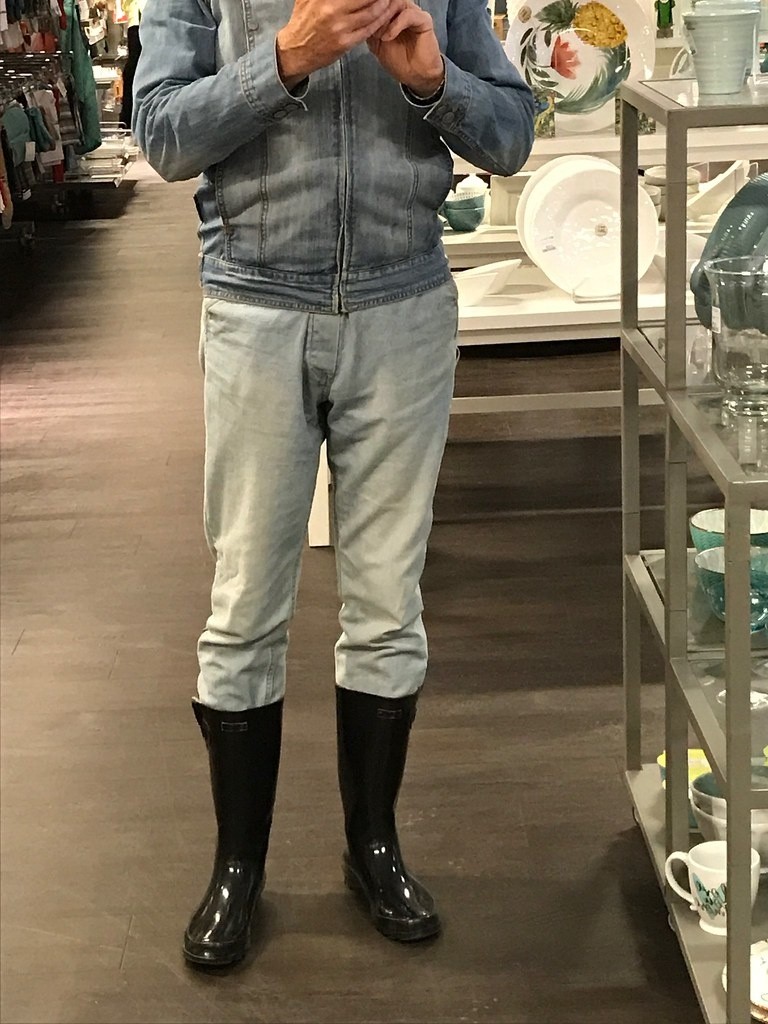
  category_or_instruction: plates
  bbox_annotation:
[689,159,750,218]
[506,0,656,133]
[515,155,659,297]
[722,938,768,1024]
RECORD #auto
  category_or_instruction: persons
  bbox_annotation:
[130,0,536,966]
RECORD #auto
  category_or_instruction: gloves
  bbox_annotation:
[1,107,59,167]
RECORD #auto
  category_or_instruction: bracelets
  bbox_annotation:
[407,75,445,101]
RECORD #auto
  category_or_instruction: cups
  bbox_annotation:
[682,0,768,95]
[705,255,768,394]
[664,840,760,935]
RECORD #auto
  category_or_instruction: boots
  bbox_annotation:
[181,695,285,966]
[335,681,444,939]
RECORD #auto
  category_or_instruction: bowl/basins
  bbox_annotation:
[656,749,768,884]
[641,167,701,221]
[444,189,484,232]
[454,258,523,308]
[690,506,768,634]
[656,231,708,283]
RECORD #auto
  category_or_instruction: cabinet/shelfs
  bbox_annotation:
[610,74,768,1021]
